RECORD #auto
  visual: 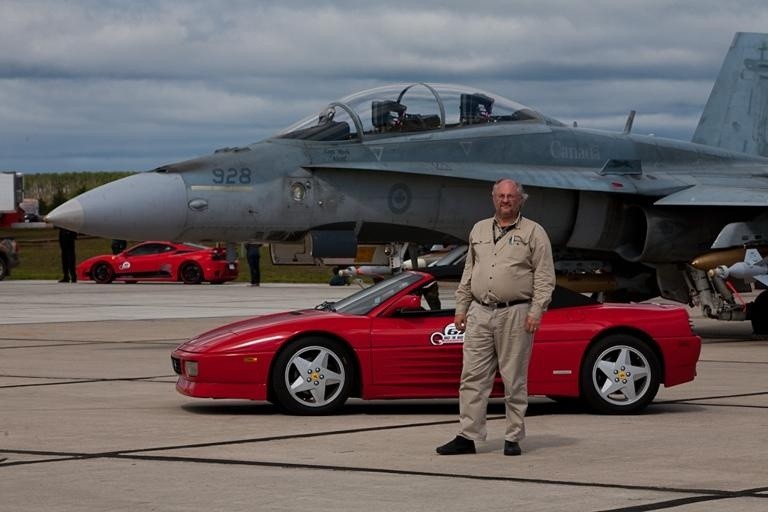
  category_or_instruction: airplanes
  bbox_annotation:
[42,30,768,342]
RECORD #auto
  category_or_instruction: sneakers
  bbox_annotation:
[505,439,521,455]
[435,435,476,456]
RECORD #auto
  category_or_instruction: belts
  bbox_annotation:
[470,294,531,310]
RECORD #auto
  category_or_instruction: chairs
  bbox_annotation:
[460,93,495,125]
[372,100,407,134]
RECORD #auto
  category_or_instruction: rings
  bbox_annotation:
[533,327,537,330]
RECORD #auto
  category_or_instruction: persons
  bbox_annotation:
[245,243,261,286]
[330,265,351,287]
[53,225,79,283]
[110,238,127,256]
[434,175,556,458]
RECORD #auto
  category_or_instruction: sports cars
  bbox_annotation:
[168,271,704,414]
[72,241,240,286]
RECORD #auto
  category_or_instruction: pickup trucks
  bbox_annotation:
[0,236,20,281]
